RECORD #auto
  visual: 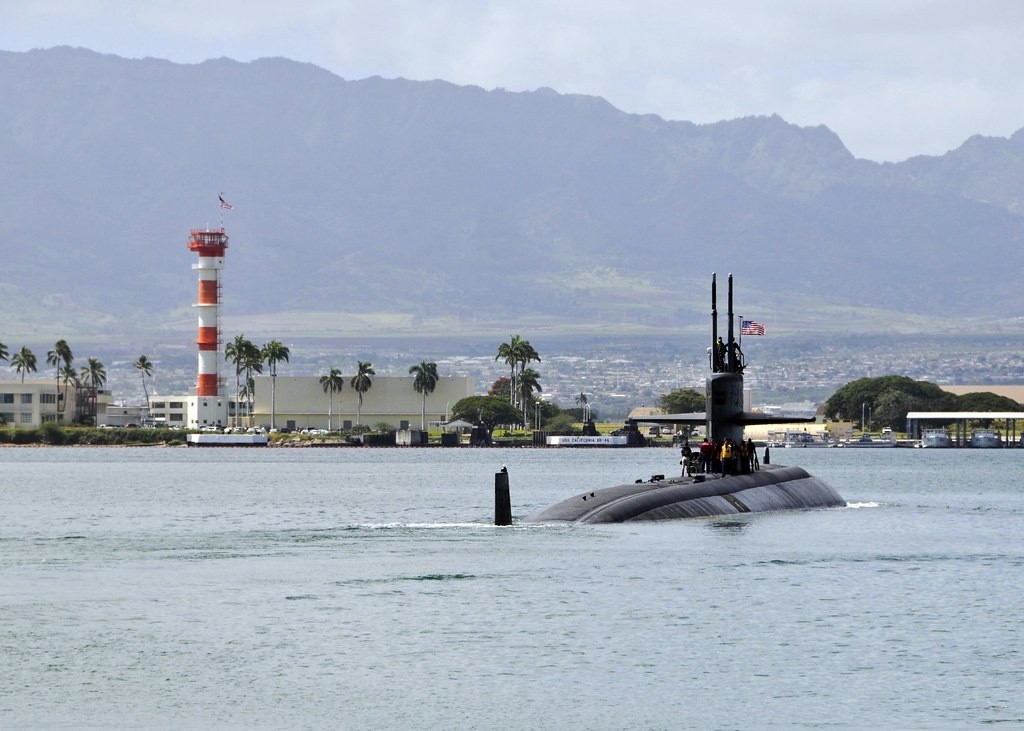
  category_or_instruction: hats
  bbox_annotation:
[704,438,708,442]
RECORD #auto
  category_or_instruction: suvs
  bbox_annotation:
[882,426,892,433]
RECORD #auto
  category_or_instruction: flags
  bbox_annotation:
[218,195,232,209]
[741,320,766,335]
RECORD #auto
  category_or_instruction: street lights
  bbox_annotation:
[869,408,871,433]
[862,403,866,434]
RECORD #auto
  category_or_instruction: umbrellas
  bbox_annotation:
[447,419,473,436]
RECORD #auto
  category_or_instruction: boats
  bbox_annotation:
[545,403,652,449]
[765,429,785,447]
[672,428,689,448]
[969,428,1004,447]
[844,433,897,447]
[921,426,953,448]
[783,430,829,448]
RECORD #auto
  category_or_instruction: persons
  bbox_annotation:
[719,437,736,479]
[710,437,757,475]
[717,340,725,372]
[725,337,744,372]
[680,443,692,477]
[699,437,717,474]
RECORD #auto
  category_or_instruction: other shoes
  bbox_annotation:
[688,474,692,477]
[701,471,718,474]
[680,475,684,477]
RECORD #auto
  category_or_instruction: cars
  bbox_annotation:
[662,427,670,433]
[648,427,656,434]
[97,422,330,436]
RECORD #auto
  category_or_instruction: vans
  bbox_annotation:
[673,430,698,436]
[610,428,633,437]
